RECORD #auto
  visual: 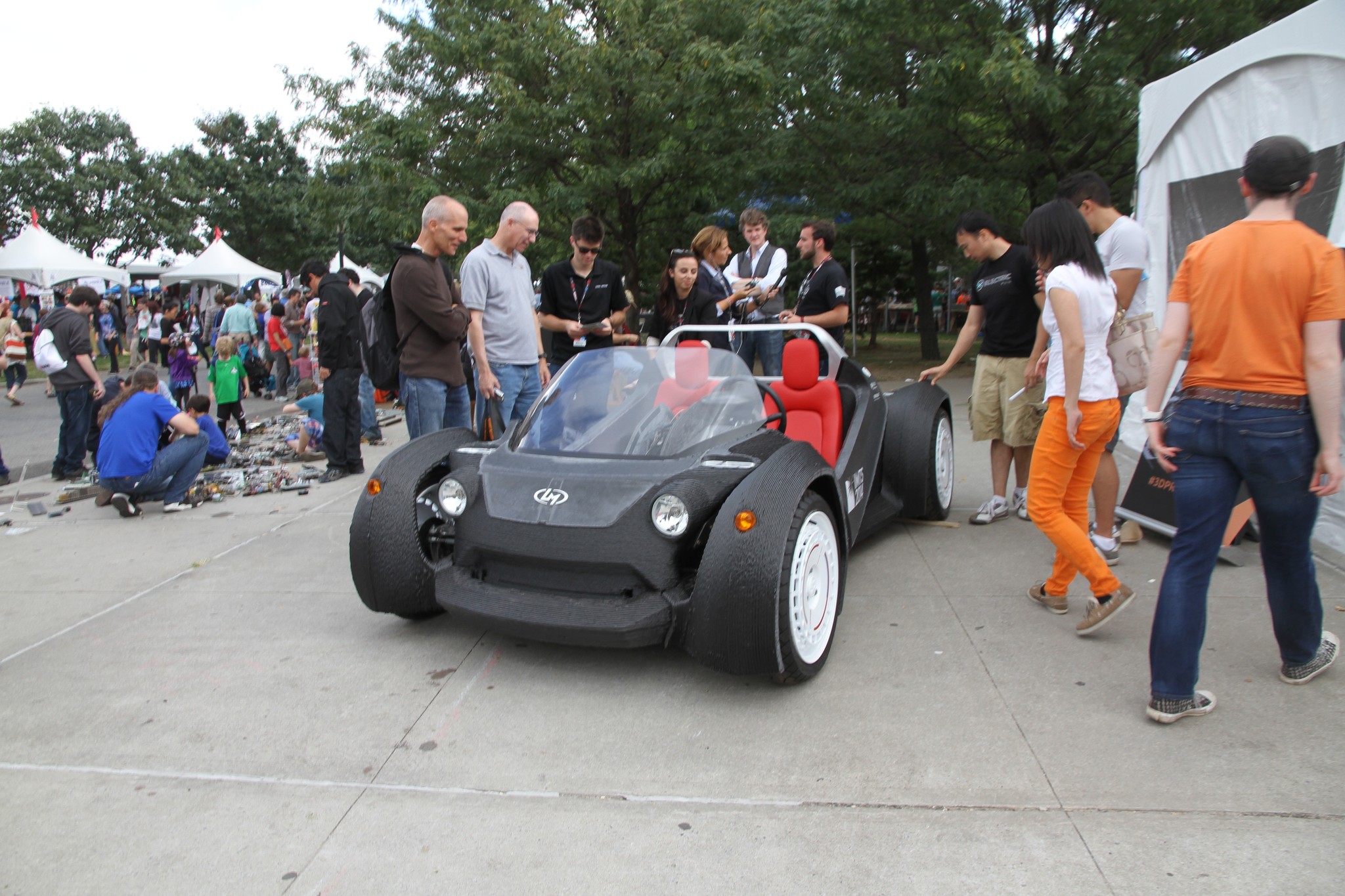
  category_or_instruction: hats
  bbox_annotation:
[1244,135,1313,192]
[290,379,316,401]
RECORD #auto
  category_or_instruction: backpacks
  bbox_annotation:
[365,238,452,390]
[32,326,67,377]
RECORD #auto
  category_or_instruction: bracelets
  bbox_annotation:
[1144,417,1163,423]
[727,297,731,307]
[1144,412,1163,418]
[801,316,811,337]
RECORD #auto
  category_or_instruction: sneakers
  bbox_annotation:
[1279,630,1340,684]
[110,493,143,516]
[969,498,1009,525]
[1075,584,1136,635]
[1087,523,1121,567]
[1146,690,1216,723]
[1010,491,1031,520]
[1027,580,1069,614]
[163,495,203,511]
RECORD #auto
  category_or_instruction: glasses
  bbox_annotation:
[670,248,696,263]
[514,220,539,236]
[574,238,603,254]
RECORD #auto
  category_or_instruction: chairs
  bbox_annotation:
[761,339,843,469]
[653,339,719,419]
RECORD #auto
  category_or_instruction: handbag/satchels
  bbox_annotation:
[478,396,505,441]
[4,321,28,360]
[210,329,219,347]
[1104,284,1160,396]
[282,337,293,351]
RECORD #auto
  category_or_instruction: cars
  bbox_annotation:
[348,322,952,687]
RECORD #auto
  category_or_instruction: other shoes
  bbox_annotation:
[201,434,384,483]
[51,467,89,479]
[95,489,113,506]
[4,388,57,406]
[274,395,289,402]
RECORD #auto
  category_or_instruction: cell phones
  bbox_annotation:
[492,386,504,402]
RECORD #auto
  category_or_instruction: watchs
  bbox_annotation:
[755,297,760,307]
[537,353,549,357]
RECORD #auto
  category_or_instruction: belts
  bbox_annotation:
[1179,386,1301,411]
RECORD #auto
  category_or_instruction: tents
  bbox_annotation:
[0,223,132,317]
[291,251,385,290]
[159,237,283,306]
[1140,0,1345,575]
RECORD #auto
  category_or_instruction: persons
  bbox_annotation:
[1140,136,1345,722]
[1027,197,1136,636]
[0,195,638,518]
[1051,169,1148,566]
[919,209,1046,525]
[690,225,778,353]
[645,248,717,349]
[779,220,850,376]
[723,208,787,377]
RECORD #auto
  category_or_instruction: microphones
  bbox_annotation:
[764,267,789,303]
